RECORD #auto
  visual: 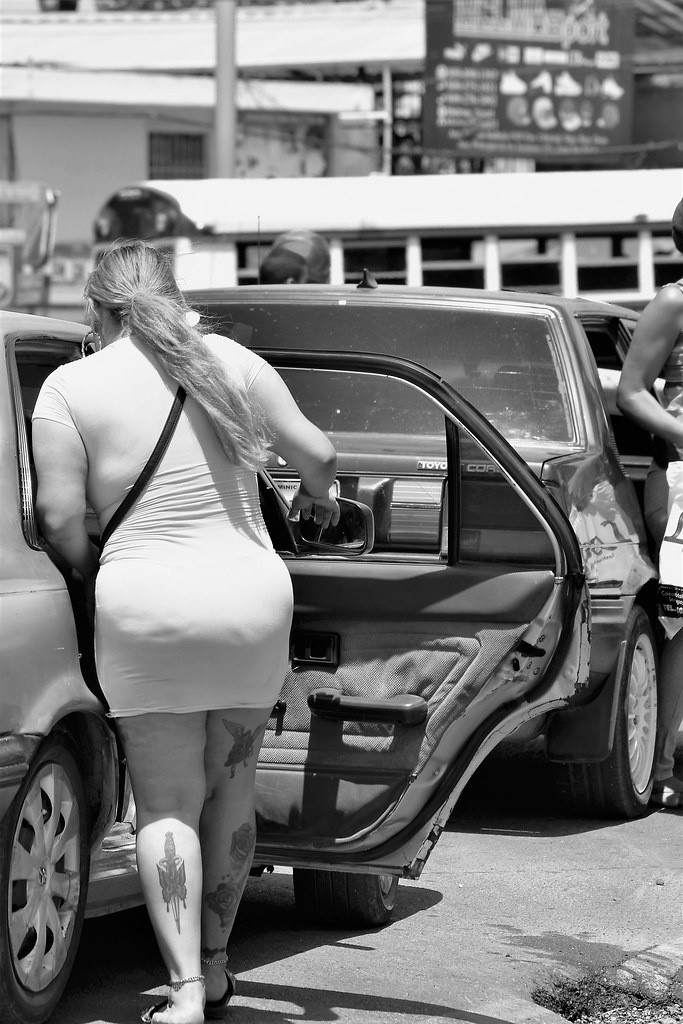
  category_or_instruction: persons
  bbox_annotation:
[259,247,308,285]
[29,244,340,1024]
[615,198,683,808]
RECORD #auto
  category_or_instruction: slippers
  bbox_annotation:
[140,999,168,1024]
[205,967,236,1018]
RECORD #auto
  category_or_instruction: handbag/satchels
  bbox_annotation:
[569,481,658,598]
[658,460,683,587]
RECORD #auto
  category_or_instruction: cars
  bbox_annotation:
[179,282,674,821]
[1,307,592,1024]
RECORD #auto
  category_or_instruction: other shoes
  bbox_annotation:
[650,778,683,806]
